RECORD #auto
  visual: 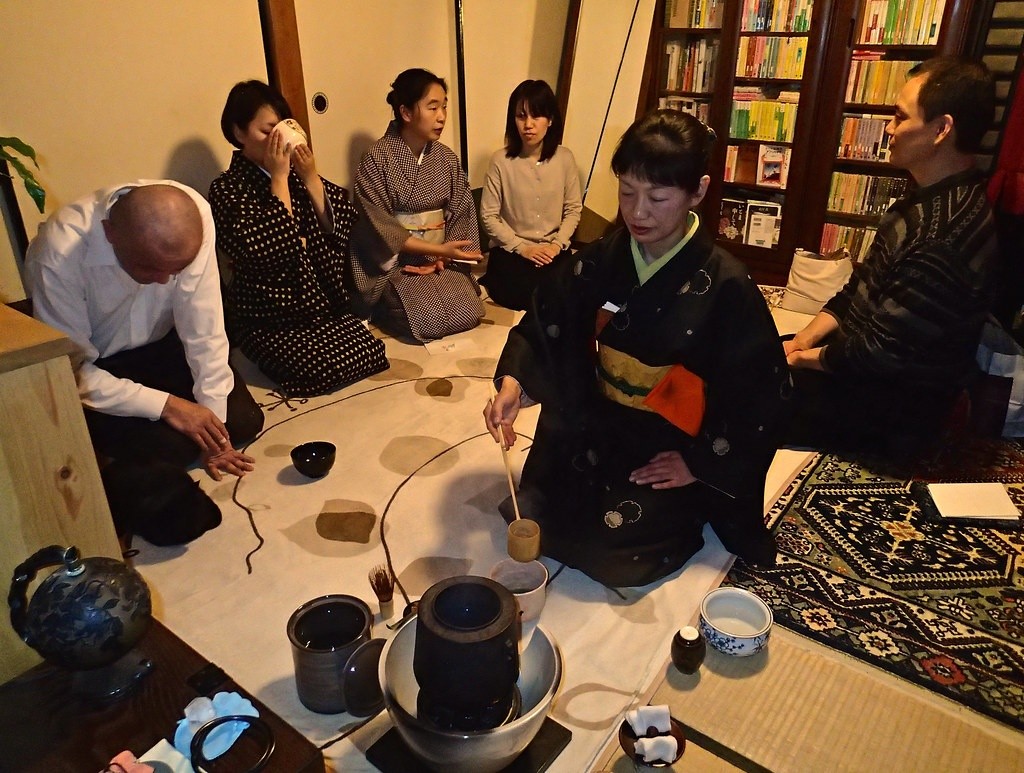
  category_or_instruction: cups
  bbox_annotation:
[286,594,373,715]
[489,557,548,654]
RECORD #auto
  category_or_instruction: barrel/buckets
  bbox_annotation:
[377,612,565,773]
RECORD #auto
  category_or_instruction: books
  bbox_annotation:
[660,0,946,263]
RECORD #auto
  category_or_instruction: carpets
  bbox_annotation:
[718,434,1024,739]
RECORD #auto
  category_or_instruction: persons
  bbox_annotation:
[484,109,788,589]
[351,68,486,342]
[26,179,265,547]
[775,53,998,480]
[479,80,584,311]
[210,80,392,397]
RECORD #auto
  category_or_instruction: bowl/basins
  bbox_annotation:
[698,587,774,658]
[619,718,686,769]
[270,118,308,164]
[290,441,337,479]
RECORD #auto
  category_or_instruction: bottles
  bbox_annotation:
[671,625,706,676]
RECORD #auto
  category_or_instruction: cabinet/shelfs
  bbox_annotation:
[647,0,834,266]
[798,0,977,274]
[0,301,125,685]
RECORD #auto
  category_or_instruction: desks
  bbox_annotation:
[0,617,326,773]
